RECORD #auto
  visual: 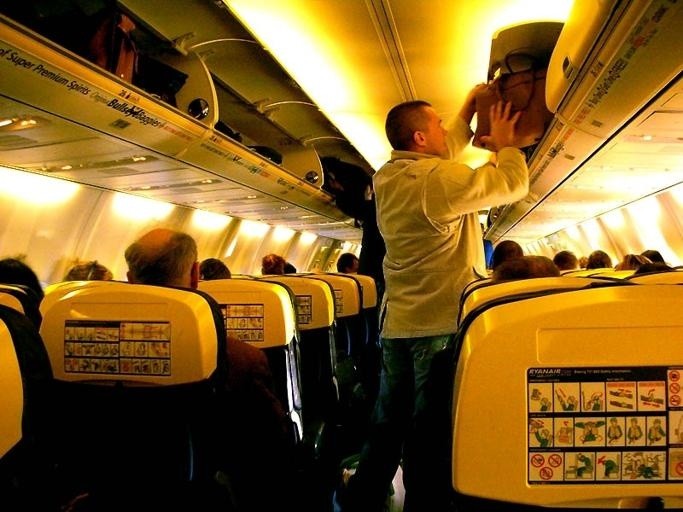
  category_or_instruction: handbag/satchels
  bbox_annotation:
[0,0,187,108]
[470,51,555,151]
[216,120,284,164]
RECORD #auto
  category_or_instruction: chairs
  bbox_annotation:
[0,268,683,512]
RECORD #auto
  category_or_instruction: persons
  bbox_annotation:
[487,240,675,284]
[1,259,46,302]
[63,261,112,281]
[331,82,533,508]
[337,253,360,276]
[125,229,297,487]
[327,179,385,307]
